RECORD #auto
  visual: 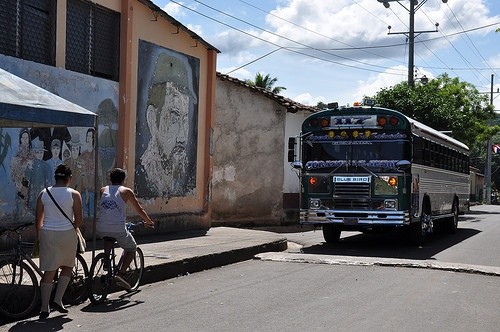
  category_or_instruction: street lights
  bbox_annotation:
[407,75,429,120]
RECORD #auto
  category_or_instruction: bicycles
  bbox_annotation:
[0,221,89,320]
[86,219,154,306]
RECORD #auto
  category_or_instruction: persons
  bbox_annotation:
[36,164,84,320]
[96,168,153,289]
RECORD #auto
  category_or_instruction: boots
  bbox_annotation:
[38,281,53,320]
[52,275,70,313]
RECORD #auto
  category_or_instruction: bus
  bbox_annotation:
[287,101,471,246]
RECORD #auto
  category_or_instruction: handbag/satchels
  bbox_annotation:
[75,225,86,254]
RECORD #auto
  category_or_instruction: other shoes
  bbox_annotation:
[102,262,115,271]
[114,272,131,291]
